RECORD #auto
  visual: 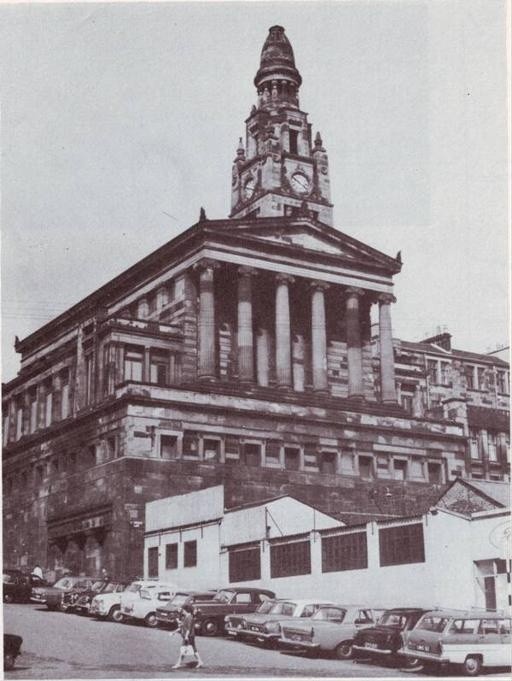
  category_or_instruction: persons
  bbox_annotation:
[169,603,204,670]
[96,567,112,594]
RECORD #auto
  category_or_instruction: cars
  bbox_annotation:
[349,607,441,668]
[190,587,276,637]
[394,607,512,676]
[3,567,188,630]
[223,599,333,648]
[154,590,215,627]
[277,604,390,659]
